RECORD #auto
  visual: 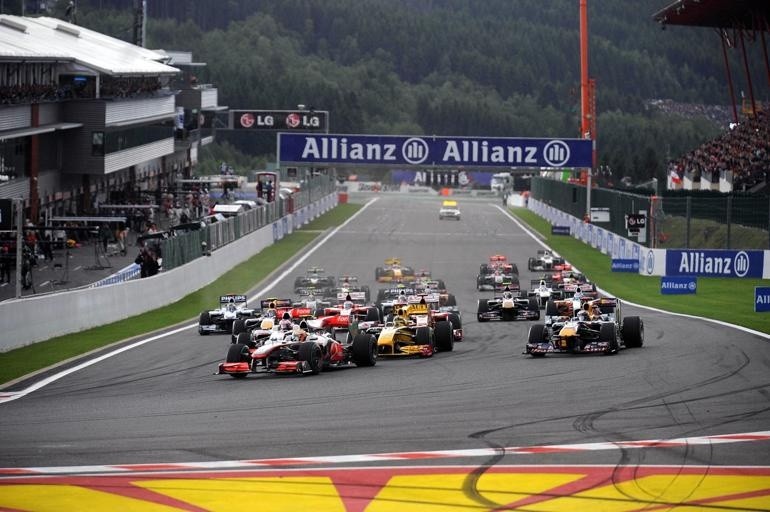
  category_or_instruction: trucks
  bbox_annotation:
[489,171,534,195]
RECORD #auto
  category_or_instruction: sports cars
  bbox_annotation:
[439,200,461,220]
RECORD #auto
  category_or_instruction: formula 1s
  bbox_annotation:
[472,251,645,358]
[193,256,465,376]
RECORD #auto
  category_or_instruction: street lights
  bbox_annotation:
[296,101,315,182]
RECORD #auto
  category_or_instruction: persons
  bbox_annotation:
[0,177,212,282]
[266,180,273,202]
[665,101,770,188]
[255,180,263,198]
